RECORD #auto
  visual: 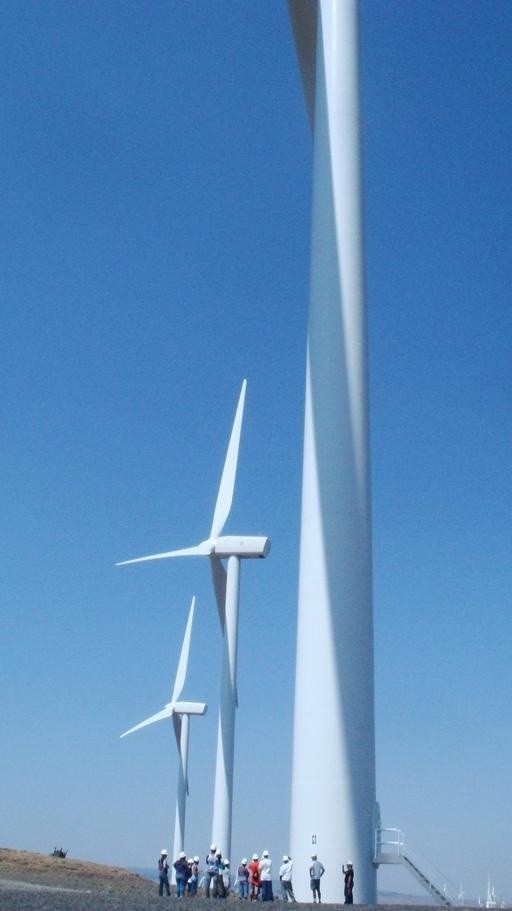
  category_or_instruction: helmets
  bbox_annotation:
[312,853,317,858]
[283,856,289,862]
[242,851,269,865]
[211,845,230,866]
[180,852,199,863]
[347,861,354,866]
[161,850,168,855]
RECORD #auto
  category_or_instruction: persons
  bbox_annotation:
[248,853,260,902]
[279,856,297,903]
[158,849,171,896]
[236,858,249,901]
[342,860,354,904]
[191,856,199,896]
[309,854,325,903]
[198,843,231,899]
[187,858,194,897]
[257,850,274,901]
[173,852,192,898]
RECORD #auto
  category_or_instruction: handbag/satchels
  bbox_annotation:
[253,872,258,880]
